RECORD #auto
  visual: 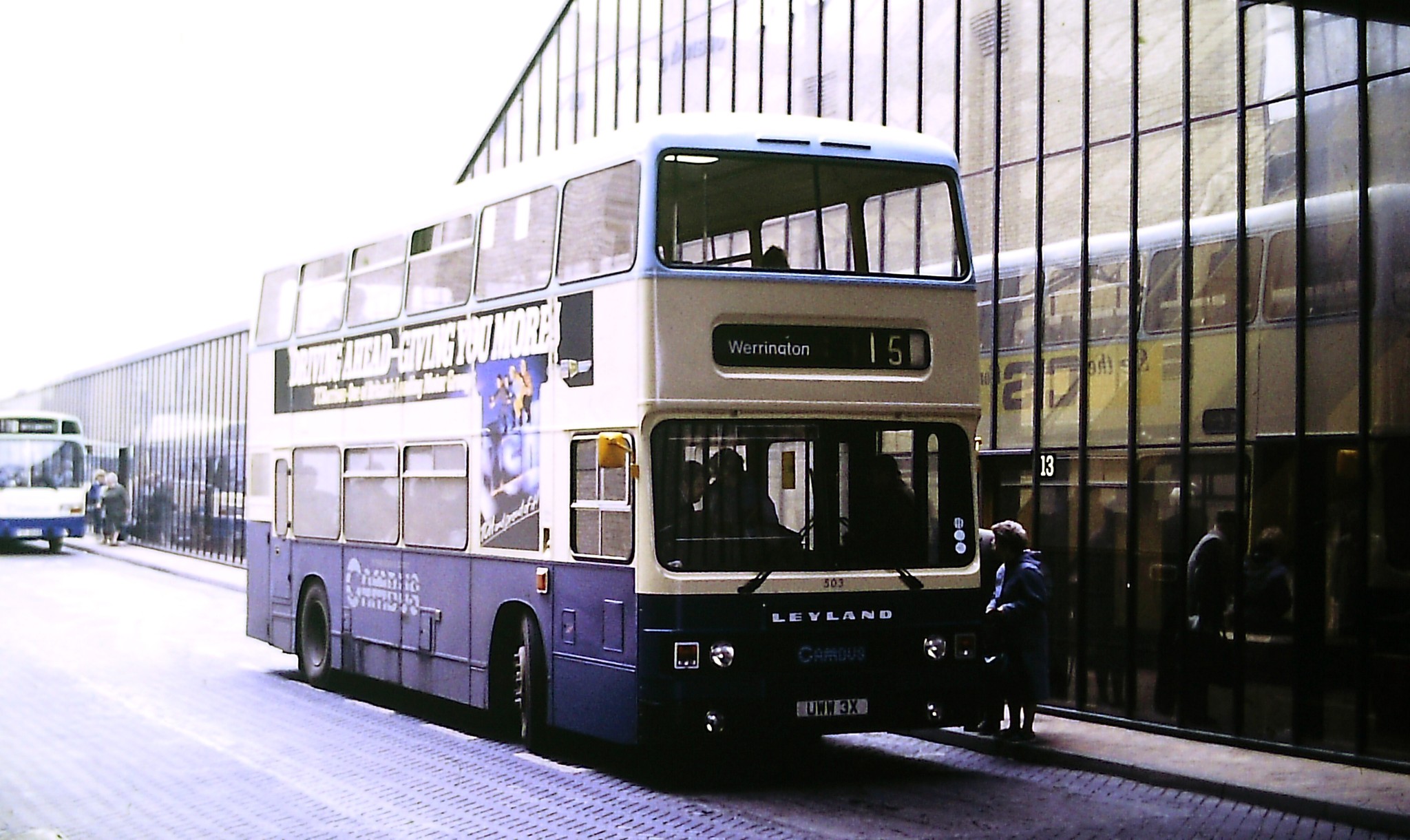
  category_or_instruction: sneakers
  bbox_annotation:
[995,727,1022,736]
[1009,730,1036,744]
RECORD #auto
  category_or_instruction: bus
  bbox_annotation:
[876,182,1410,700]
[128,415,246,557]
[0,410,87,554]
[243,111,995,758]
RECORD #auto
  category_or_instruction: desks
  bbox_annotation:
[1219,624,1357,740]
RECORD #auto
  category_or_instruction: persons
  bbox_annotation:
[502,375,517,431]
[972,526,1008,736]
[507,364,525,428]
[488,374,511,435]
[669,457,712,540]
[759,245,794,270]
[88,469,112,543]
[97,471,132,547]
[518,357,535,425]
[983,519,1048,742]
[1183,506,1236,726]
[705,444,780,540]
[864,450,939,567]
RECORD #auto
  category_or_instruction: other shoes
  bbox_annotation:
[963,719,982,731]
[980,722,999,735]
